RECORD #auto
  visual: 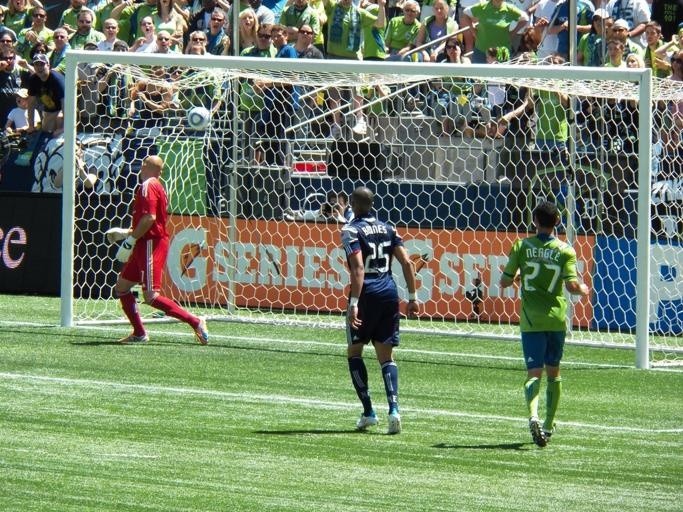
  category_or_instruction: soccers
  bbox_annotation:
[188,105,211,131]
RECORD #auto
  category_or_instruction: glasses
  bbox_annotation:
[2,11,314,62]
[612,27,628,32]
[404,8,418,15]
[444,44,458,49]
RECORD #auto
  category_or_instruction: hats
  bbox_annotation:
[13,89,32,100]
[32,53,50,66]
[591,6,608,21]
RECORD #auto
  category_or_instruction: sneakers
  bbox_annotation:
[351,120,368,135]
[325,124,342,141]
[195,317,209,345]
[390,414,402,434]
[528,419,555,446]
[115,334,151,343]
[354,414,379,429]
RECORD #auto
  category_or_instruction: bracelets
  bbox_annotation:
[349,296,359,307]
[408,292,417,301]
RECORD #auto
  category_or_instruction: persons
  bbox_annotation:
[104,156,208,345]
[500,202,590,447]
[339,186,420,435]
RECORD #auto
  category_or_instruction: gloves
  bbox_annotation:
[106,227,128,244]
[116,237,137,263]
